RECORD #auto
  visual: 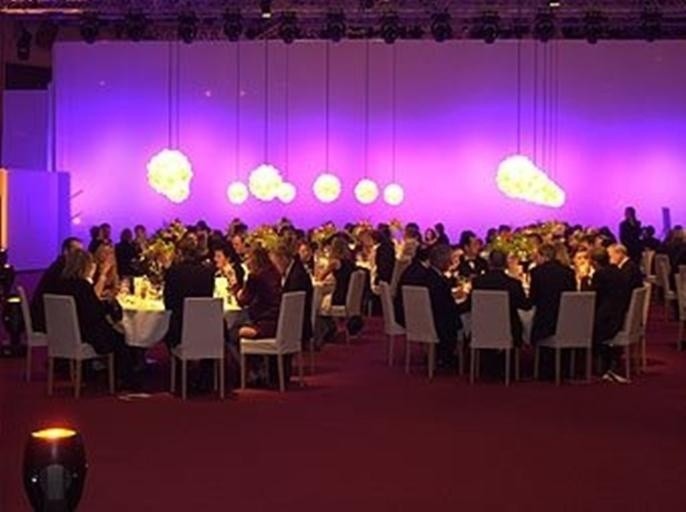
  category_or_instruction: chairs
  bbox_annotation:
[16,247,366,401]
[369,249,685,391]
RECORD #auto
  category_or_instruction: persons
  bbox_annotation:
[618,205,644,262]
[29,216,363,395]
[344,218,643,379]
[641,224,686,320]
